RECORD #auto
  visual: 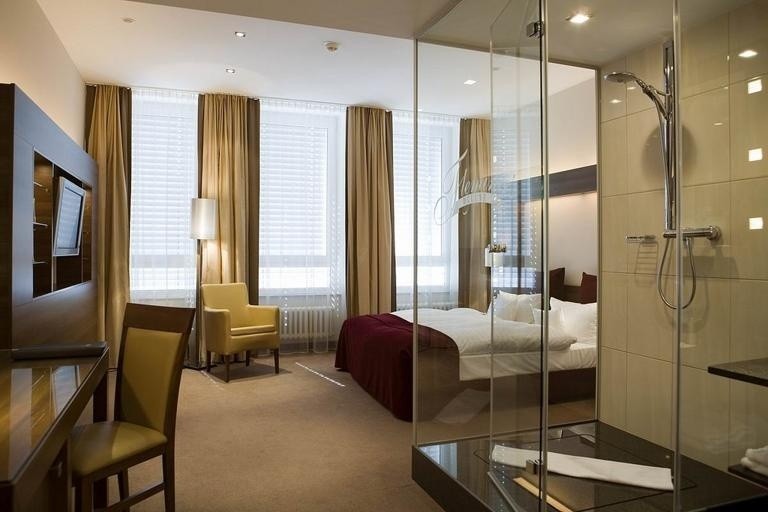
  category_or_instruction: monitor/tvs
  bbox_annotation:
[53,176,86,257]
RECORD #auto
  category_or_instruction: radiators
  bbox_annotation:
[279,307,335,342]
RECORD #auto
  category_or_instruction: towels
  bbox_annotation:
[741,445,768,475]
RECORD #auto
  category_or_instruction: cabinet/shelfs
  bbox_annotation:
[0,83,99,348]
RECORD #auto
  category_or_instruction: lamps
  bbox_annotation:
[184,198,217,370]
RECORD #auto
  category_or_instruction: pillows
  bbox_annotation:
[496,268,597,345]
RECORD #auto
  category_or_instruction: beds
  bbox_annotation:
[335,285,597,423]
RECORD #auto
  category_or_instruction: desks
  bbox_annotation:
[412,422,768,512]
[0,342,109,512]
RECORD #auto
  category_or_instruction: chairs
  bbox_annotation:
[70,302,196,512]
[200,283,280,382]
